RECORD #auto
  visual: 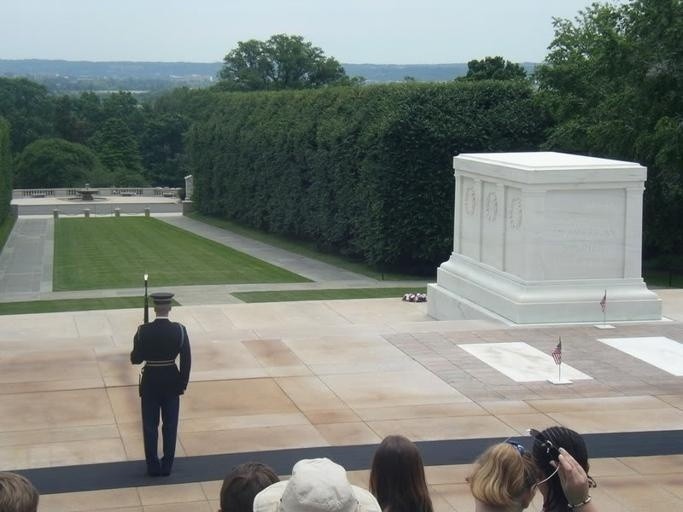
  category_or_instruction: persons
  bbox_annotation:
[220,457,382,512]
[0,470,37,512]
[369,435,433,512]
[467,425,594,512]
[128,290,191,481]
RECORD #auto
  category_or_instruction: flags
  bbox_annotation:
[598,289,612,316]
[551,343,565,368]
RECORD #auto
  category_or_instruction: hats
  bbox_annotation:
[252,457,382,512]
[149,294,173,302]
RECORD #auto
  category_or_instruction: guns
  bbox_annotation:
[139,269,148,397]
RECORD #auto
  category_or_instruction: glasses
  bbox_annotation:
[507,437,524,457]
